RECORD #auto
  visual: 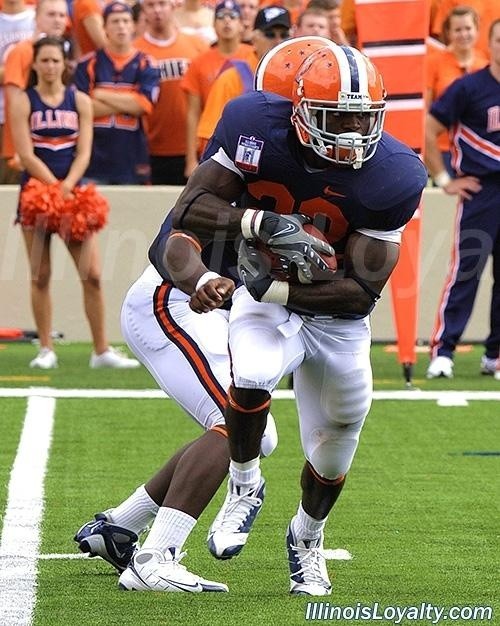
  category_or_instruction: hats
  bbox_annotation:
[215,1,241,15]
[252,6,291,31]
[102,0,134,17]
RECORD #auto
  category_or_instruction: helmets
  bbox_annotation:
[255,35,338,105]
[292,46,386,169]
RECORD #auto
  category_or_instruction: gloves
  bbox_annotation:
[241,209,335,280]
[235,234,289,307]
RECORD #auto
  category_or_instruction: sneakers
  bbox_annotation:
[89,347,141,370]
[118,542,229,593]
[287,515,332,597]
[426,356,454,379]
[74,512,140,574]
[480,355,500,380]
[207,477,266,560]
[28,348,59,370]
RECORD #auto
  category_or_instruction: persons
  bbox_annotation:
[133,1,207,186]
[0,0,34,177]
[71,1,106,57]
[174,3,215,43]
[3,0,69,176]
[10,35,141,369]
[310,0,349,47]
[196,5,291,153]
[168,47,428,595]
[72,36,338,592]
[424,17,500,379]
[428,9,489,181]
[180,1,251,182]
[236,0,256,44]
[298,9,326,40]
[73,4,160,184]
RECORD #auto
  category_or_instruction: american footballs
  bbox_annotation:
[256,223,338,282]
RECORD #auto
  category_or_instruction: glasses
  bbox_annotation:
[106,19,132,24]
[215,12,239,19]
[261,30,291,39]
